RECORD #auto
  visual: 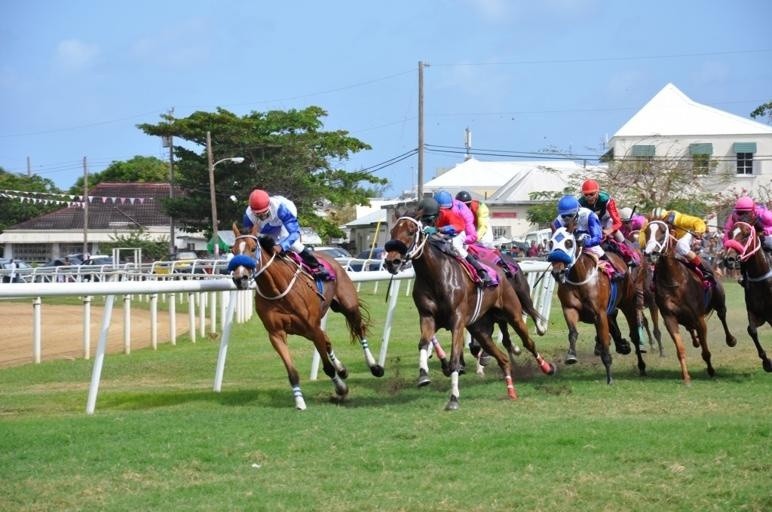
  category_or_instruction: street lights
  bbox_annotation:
[207,157,246,249]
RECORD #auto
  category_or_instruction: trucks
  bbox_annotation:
[524,227,552,247]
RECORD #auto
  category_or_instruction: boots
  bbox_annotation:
[621,239,637,268]
[496,255,513,278]
[698,262,717,285]
[465,253,492,287]
[297,246,330,277]
[599,252,623,282]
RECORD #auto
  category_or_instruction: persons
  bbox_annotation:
[414,186,552,288]
[554,193,622,283]
[690,226,740,283]
[723,197,772,283]
[619,208,649,244]
[579,184,636,267]
[640,206,710,290]
[242,190,331,283]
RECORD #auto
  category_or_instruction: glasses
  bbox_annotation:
[584,193,596,196]
[561,214,575,218]
[420,216,436,222]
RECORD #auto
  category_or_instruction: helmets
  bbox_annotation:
[419,198,438,216]
[618,207,636,222]
[455,191,472,204]
[734,196,755,213]
[652,207,667,220]
[582,180,599,194]
[558,194,579,215]
[433,190,453,209]
[249,189,270,213]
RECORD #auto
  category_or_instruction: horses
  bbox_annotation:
[548,210,666,387]
[231,220,385,410]
[723,210,772,373]
[383,208,554,411]
[642,213,737,387]
